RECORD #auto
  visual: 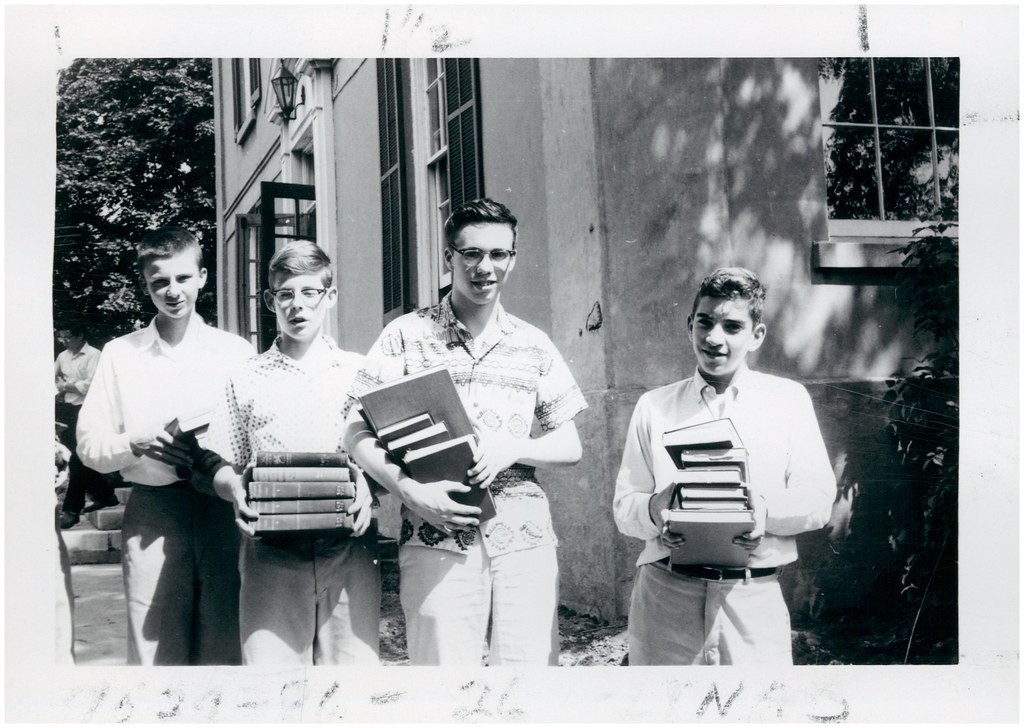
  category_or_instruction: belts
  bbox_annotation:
[659,557,776,583]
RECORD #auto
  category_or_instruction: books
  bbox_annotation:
[661,416,757,568]
[158,400,211,452]
[355,365,497,529]
[247,448,353,533]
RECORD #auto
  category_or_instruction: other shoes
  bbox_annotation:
[83,496,119,513]
[60,512,80,529]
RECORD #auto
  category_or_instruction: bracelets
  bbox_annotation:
[62,382,67,392]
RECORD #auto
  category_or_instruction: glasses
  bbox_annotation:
[274,286,326,304]
[450,242,516,266]
[58,335,74,342]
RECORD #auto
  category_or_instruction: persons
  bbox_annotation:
[77,225,259,667]
[55,322,119,529]
[202,240,381,667]
[612,267,837,666]
[343,199,589,667]
